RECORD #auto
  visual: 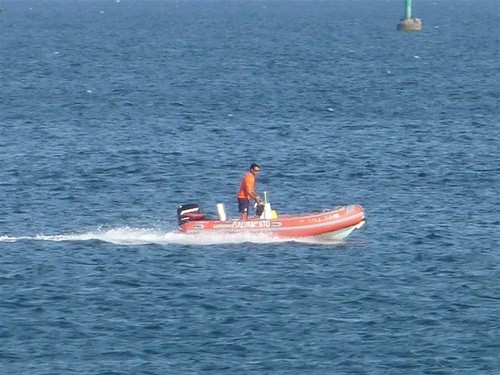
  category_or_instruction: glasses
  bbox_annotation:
[252,169,260,172]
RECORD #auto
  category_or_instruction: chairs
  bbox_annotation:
[218,203,240,222]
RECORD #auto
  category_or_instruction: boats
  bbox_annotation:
[178,191,365,242]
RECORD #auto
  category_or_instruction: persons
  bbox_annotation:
[237,163,260,221]
